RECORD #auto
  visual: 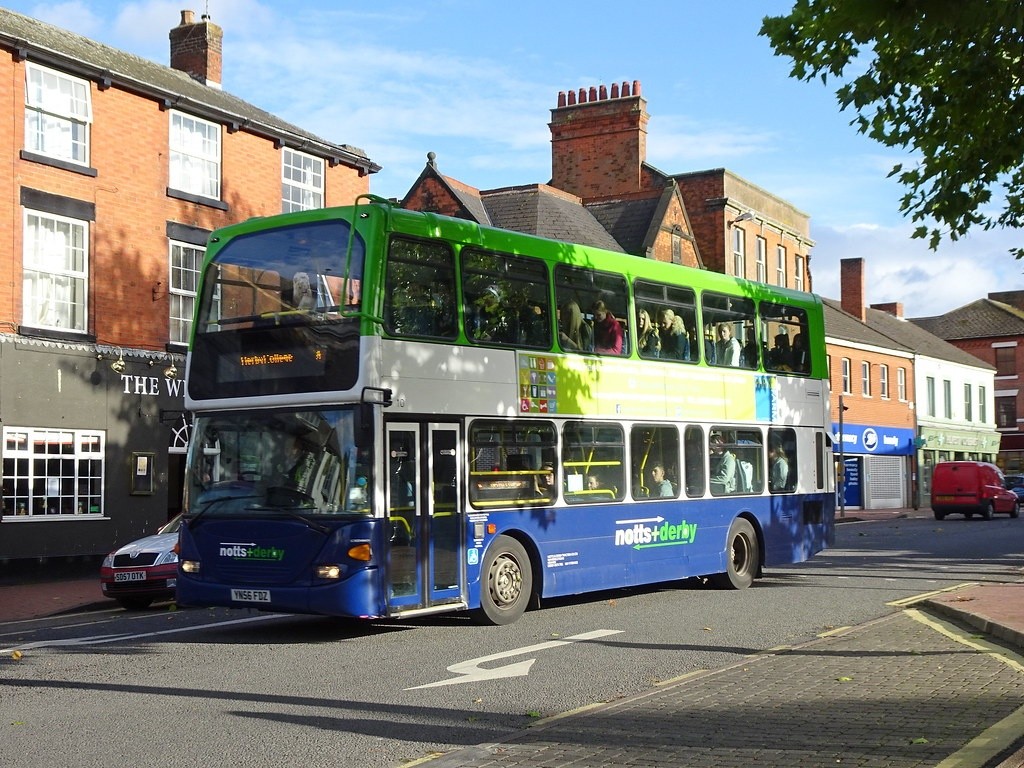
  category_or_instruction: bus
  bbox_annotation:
[178,193,837,624]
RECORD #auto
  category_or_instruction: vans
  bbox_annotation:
[931,461,1021,521]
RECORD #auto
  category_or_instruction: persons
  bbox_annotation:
[233,287,811,514]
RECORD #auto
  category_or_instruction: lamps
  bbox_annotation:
[95,341,126,375]
[149,352,177,380]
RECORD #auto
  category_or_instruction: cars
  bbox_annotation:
[1005,473,1024,504]
[101,512,185,611]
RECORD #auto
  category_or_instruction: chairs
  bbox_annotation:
[535,450,791,501]
[467,306,808,375]
